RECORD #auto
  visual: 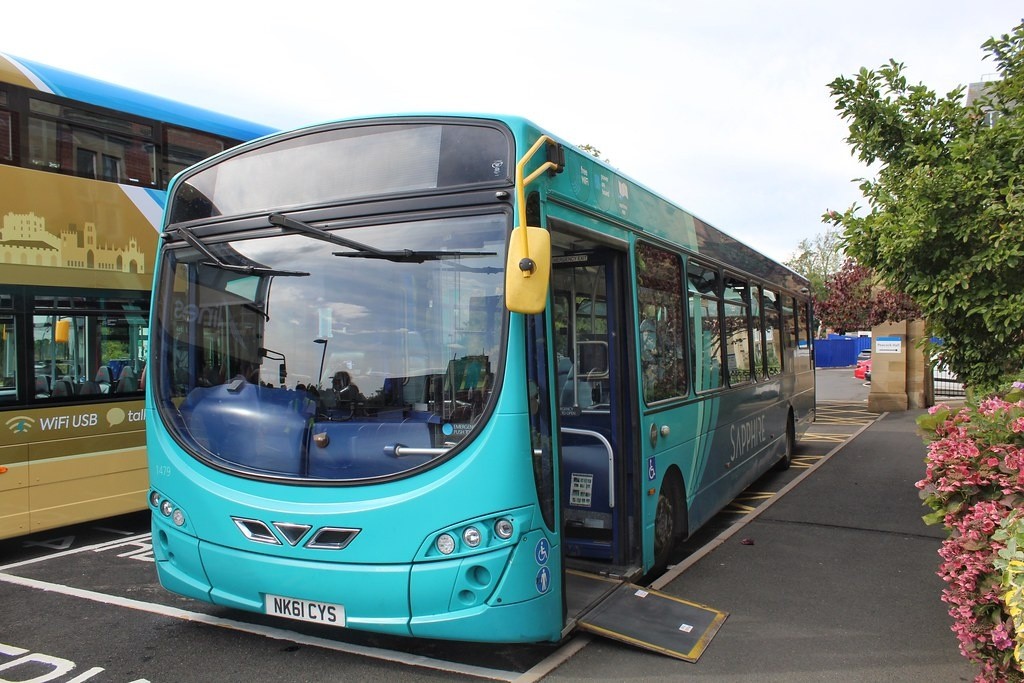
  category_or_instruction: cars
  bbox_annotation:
[855,359,873,380]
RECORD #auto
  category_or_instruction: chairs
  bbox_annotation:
[33,366,138,398]
[558,358,595,409]
[402,331,429,404]
[640,319,656,355]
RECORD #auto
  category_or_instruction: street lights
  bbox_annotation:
[315,338,328,386]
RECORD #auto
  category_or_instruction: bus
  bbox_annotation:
[1,51,295,543]
[140,112,820,663]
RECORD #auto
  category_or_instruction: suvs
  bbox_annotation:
[858,348,872,360]
